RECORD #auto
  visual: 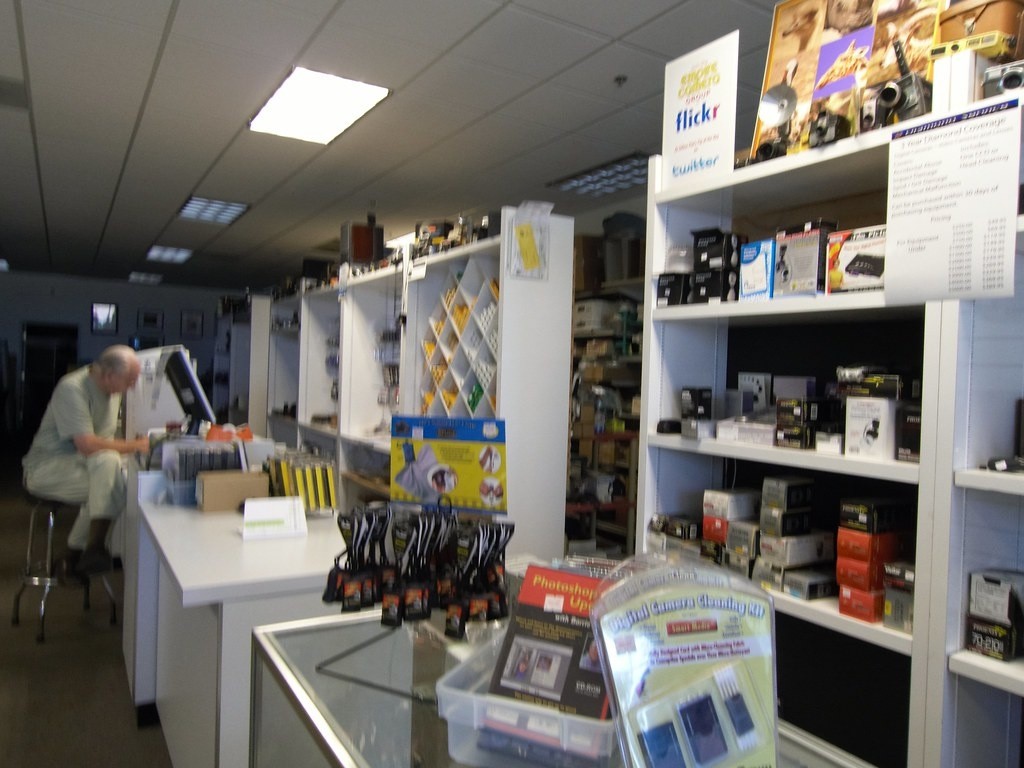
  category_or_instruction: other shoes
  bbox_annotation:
[56,559,82,585]
[80,554,110,571]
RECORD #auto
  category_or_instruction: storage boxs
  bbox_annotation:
[693,269,739,304]
[784,568,837,600]
[772,216,840,294]
[647,485,759,581]
[750,557,802,593]
[691,226,749,274]
[964,566,1024,661]
[759,505,813,538]
[758,530,835,568]
[836,497,915,633]
[760,476,816,515]
[195,469,270,514]
[656,273,693,305]
[680,370,921,464]
[435,631,620,768]
[565,211,630,467]
[163,476,195,508]
[738,239,776,299]
[824,224,885,294]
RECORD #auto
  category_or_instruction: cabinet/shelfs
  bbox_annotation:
[212,294,270,438]
[266,204,575,560]
[922,49,1024,768]
[634,56,923,768]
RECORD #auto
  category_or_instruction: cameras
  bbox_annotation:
[755,73,932,162]
[981,60,1024,99]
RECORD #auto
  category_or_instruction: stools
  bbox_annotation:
[11,494,117,645]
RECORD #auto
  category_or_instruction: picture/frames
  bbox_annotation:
[128,335,165,350]
[90,301,119,336]
[180,308,204,340]
[136,307,164,333]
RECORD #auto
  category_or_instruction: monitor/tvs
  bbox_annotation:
[161,351,215,436]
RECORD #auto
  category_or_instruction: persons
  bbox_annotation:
[22,344,150,588]
